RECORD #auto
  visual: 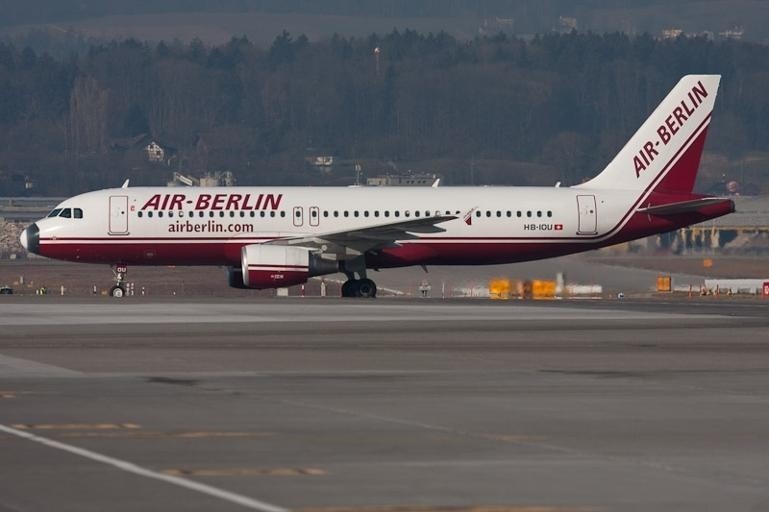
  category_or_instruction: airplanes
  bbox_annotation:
[19,74,738,301]
